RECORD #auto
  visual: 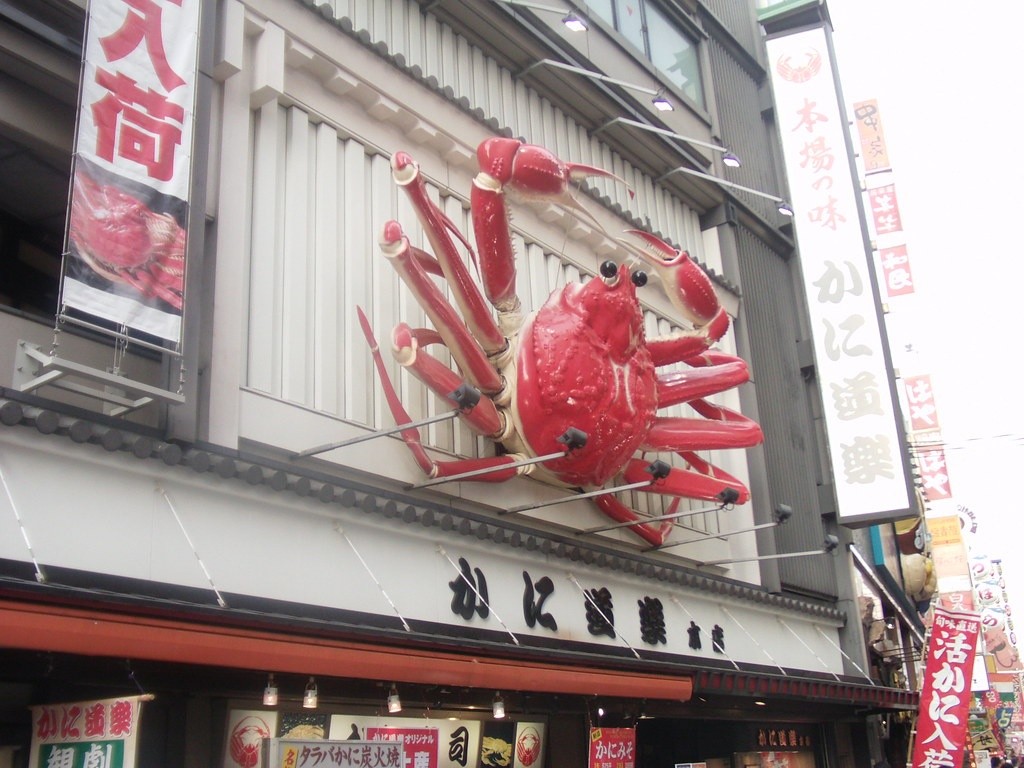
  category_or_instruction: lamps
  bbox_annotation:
[386,682,401,713]
[422,0,589,32]
[574,488,739,536]
[496,459,671,514]
[697,535,840,565]
[654,165,796,217]
[263,672,278,706]
[404,427,587,491]
[492,691,506,719]
[512,59,675,111]
[590,117,742,168]
[622,703,631,720]
[302,677,318,708]
[287,384,481,462]
[866,615,896,630]
[641,502,790,553]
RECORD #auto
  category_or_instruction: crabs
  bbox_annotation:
[356,135,764,548]
[69,169,186,311]
